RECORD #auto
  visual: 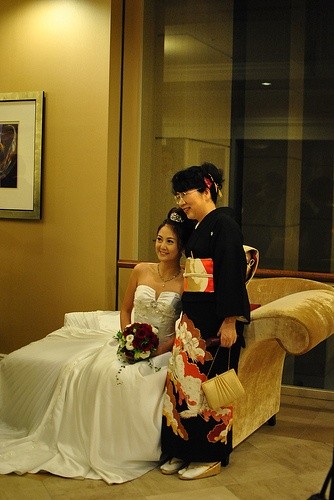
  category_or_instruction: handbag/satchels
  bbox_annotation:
[201,343,245,410]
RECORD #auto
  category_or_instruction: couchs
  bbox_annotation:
[63,277,334,467]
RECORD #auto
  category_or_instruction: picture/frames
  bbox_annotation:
[0,91,45,221]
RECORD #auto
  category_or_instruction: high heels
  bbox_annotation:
[178,461,221,480]
[160,457,190,474]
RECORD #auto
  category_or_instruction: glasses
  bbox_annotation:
[174,188,201,201]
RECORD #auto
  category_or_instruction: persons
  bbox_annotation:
[89,208,184,485]
[161,162,250,480]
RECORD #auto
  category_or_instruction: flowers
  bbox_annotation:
[113,322,162,386]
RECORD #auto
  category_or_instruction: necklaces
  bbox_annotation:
[158,263,180,287]
